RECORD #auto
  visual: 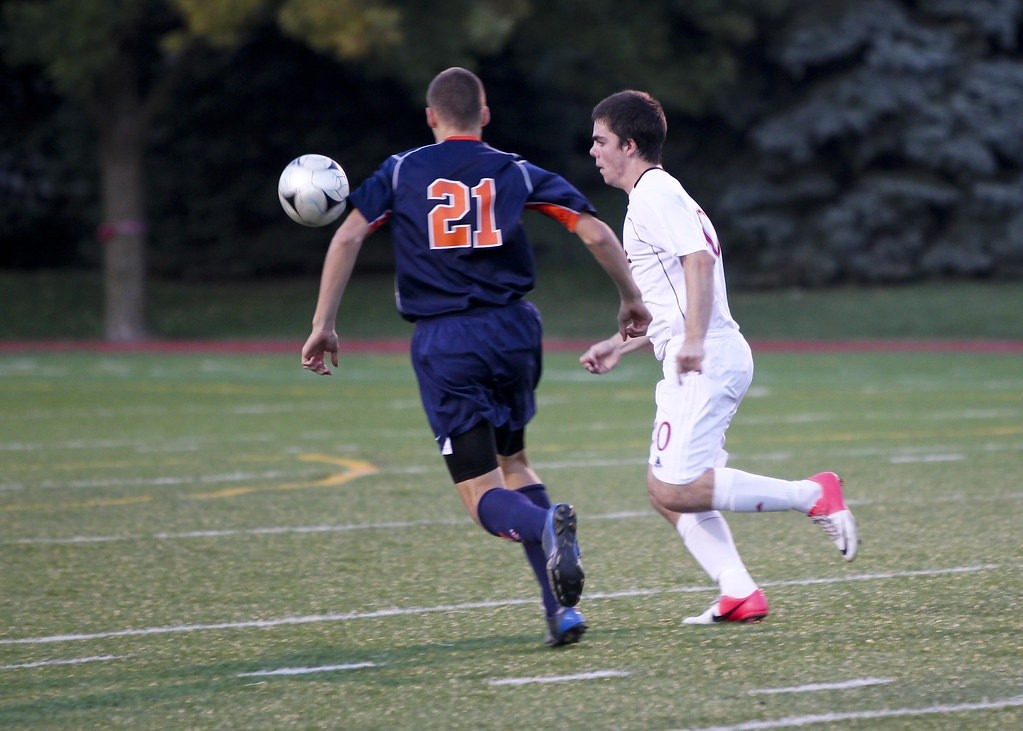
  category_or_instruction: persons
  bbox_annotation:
[302,67,652,649]
[576,91,858,623]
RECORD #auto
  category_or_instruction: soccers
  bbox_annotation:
[278,153,351,228]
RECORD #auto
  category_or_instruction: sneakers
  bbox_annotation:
[806,470,862,562]
[550,610,588,647]
[683,588,768,625]
[543,502,585,607]
